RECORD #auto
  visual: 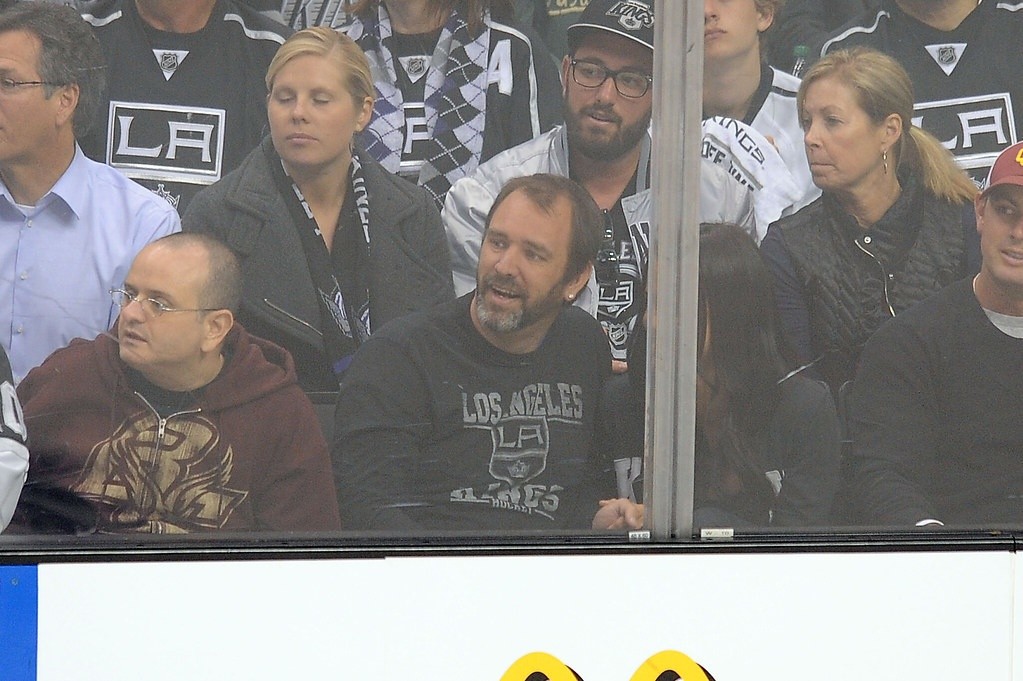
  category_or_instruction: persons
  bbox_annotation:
[852,141,1023,529]
[75,1,298,220]
[336,0,557,215]
[16,231,341,534]
[757,46,984,407]
[789,0,1023,193]
[589,223,843,532]
[182,27,455,400]
[699,0,823,245]
[330,173,613,534]
[0,0,182,389]
[440,0,757,376]
[0,342,31,536]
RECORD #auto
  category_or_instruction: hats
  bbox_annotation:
[978,140,1023,200]
[566,0,654,50]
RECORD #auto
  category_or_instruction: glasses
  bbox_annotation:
[108,289,221,318]
[570,53,653,99]
[0,78,71,92]
